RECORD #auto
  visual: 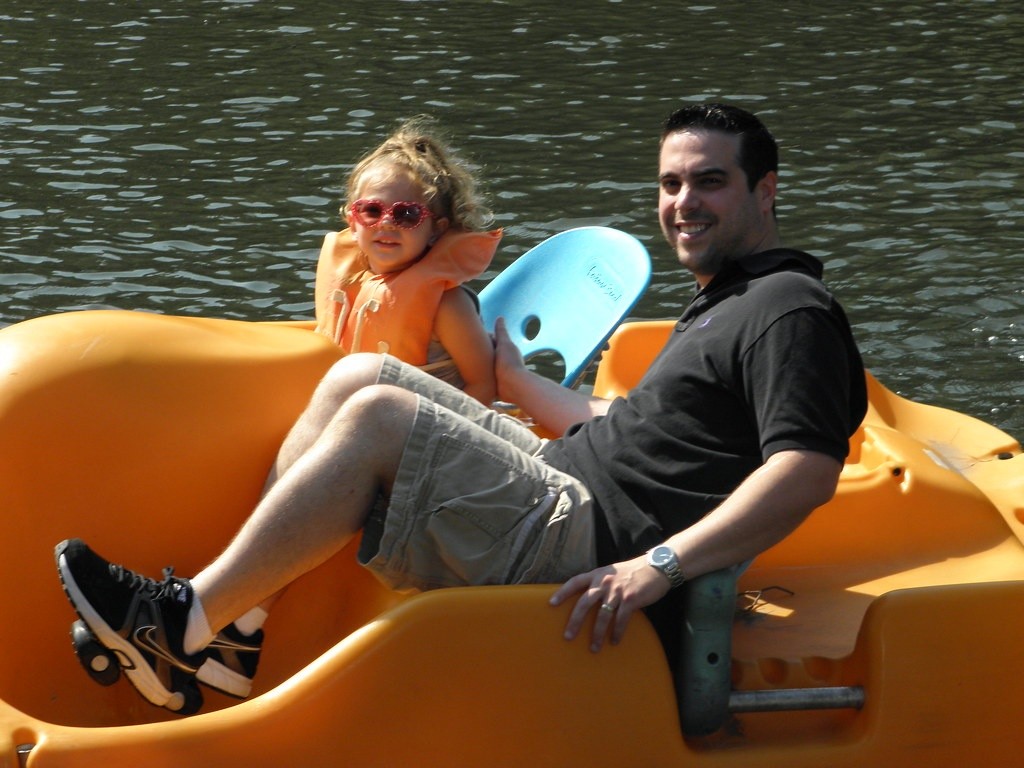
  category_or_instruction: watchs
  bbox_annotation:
[646,544,686,590]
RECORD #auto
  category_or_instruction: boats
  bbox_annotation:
[1,228,1024,767]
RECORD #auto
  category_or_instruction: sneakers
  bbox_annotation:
[195,619,266,699]
[53,539,210,707]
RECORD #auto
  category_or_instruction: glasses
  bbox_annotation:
[349,198,437,231]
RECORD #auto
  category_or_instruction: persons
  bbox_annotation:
[53,103,869,710]
[311,115,499,407]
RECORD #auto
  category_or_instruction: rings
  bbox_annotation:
[599,602,615,613]
